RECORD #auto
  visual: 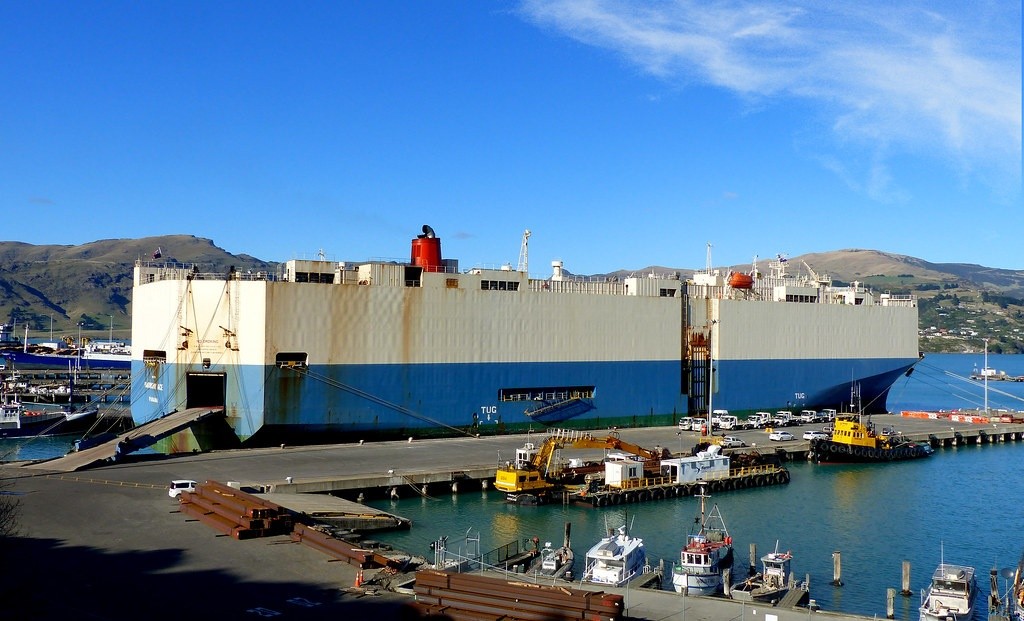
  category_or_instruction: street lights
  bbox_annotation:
[76,322,84,370]
[982,337,990,414]
[706,317,721,437]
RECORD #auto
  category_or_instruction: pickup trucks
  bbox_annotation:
[679,408,837,432]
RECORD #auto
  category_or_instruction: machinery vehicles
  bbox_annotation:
[493,424,672,502]
[806,368,937,463]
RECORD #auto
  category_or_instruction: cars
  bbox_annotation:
[720,436,746,448]
[769,430,794,441]
[803,430,829,441]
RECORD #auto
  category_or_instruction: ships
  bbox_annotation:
[20,225,924,476]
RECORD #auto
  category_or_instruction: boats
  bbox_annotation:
[918,540,977,621]
[1,374,92,395]
[1012,552,1024,621]
[0,322,132,369]
[0,322,14,359]
[729,539,802,603]
[526,522,575,578]
[672,482,734,596]
[0,400,99,438]
[581,508,645,587]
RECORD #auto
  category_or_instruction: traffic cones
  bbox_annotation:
[354,570,364,589]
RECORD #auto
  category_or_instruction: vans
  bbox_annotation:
[0,365,5,372]
[168,479,198,502]
